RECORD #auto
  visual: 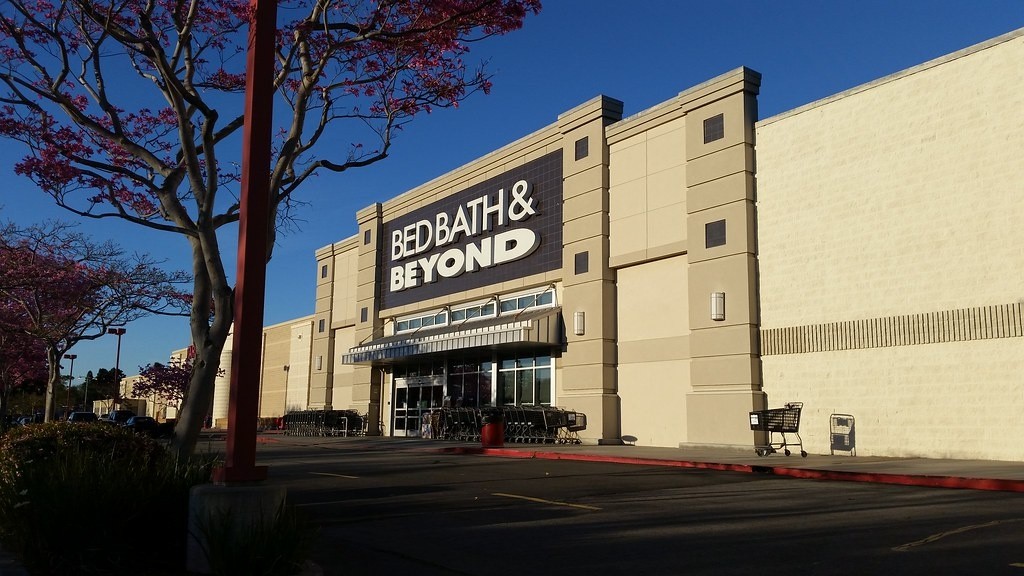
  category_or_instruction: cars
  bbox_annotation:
[123,416,159,438]
[12,416,33,425]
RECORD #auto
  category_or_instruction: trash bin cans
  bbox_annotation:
[481,409,504,447]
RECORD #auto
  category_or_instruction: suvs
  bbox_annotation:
[66,411,98,424]
[107,410,136,426]
[97,414,109,423]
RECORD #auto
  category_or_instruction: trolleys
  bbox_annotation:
[750,403,807,457]
[283,410,361,438]
[258,417,283,431]
[430,407,580,445]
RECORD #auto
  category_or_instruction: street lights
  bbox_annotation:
[107,328,126,409]
[64,354,76,412]
